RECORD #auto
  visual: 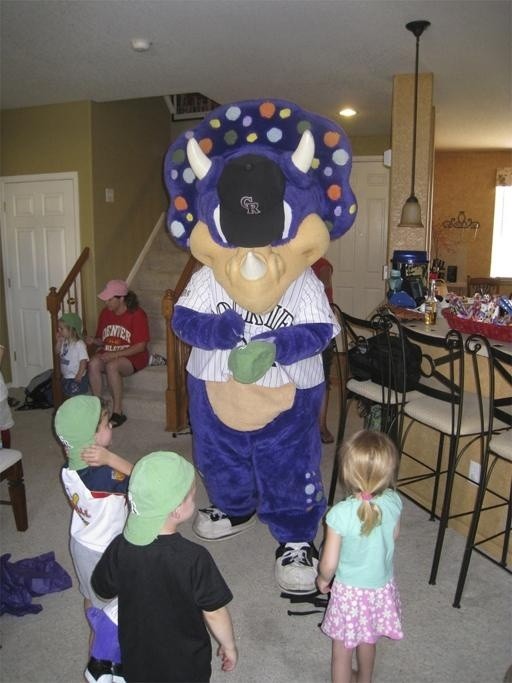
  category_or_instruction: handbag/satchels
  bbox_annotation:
[349,333,422,394]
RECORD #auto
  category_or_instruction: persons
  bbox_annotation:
[89,446,237,683]
[49,314,93,394]
[315,423,404,682]
[53,391,136,678]
[86,277,150,430]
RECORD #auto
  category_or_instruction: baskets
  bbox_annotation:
[442,306,512,343]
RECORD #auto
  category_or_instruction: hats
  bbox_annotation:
[229,342,276,385]
[97,279,130,302]
[123,452,196,546]
[216,148,288,247]
[54,396,102,472]
[62,313,86,342]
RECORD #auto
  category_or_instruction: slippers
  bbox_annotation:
[320,428,333,442]
[108,413,126,428]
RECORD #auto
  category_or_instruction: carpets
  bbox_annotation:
[0,226,512,683]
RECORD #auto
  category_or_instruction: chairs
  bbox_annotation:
[378,315,512,584]
[324,303,445,522]
[452,334,512,608]
[467,276,501,297]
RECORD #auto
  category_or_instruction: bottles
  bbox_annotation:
[424,274,438,325]
[388,271,403,299]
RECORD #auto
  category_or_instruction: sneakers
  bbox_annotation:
[82,656,116,683]
[276,542,320,597]
[194,505,260,542]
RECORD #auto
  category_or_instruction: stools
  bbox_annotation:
[0,447,28,531]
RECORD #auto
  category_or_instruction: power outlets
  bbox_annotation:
[467,460,481,487]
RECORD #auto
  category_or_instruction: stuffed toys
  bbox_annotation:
[84,597,126,679]
[164,96,357,598]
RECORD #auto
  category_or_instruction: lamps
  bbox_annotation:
[397,19,432,228]
